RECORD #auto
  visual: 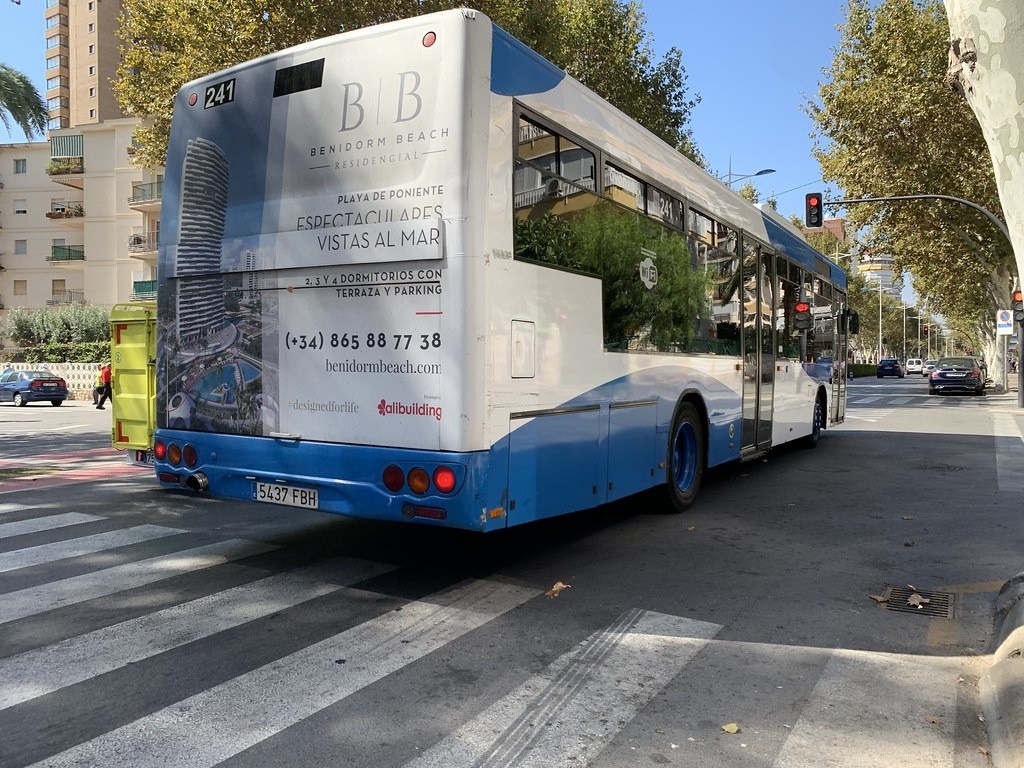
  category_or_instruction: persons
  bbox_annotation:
[0,362,13,377]
[92,363,112,409]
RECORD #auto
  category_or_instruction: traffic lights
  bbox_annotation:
[805,193,823,229]
[1012,291,1024,322]
[794,301,812,330]
[923,326,928,337]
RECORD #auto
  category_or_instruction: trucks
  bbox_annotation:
[110,291,163,470]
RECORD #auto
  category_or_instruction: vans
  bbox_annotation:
[906,359,924,375]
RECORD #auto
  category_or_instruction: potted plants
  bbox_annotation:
[45,158,83,174]
[45,203,85,219]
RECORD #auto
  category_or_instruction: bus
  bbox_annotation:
[153,4,860,533]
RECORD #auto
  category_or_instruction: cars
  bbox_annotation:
[928,357,986,396]
[0,370,68,407]
[922,359,939,377]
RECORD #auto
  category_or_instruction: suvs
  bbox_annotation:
[876,358,905,378]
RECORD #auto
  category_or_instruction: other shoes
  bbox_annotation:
[97,406,106,409]
[92,403,97,405]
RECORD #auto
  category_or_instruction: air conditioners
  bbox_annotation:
[544,177,563,194]
